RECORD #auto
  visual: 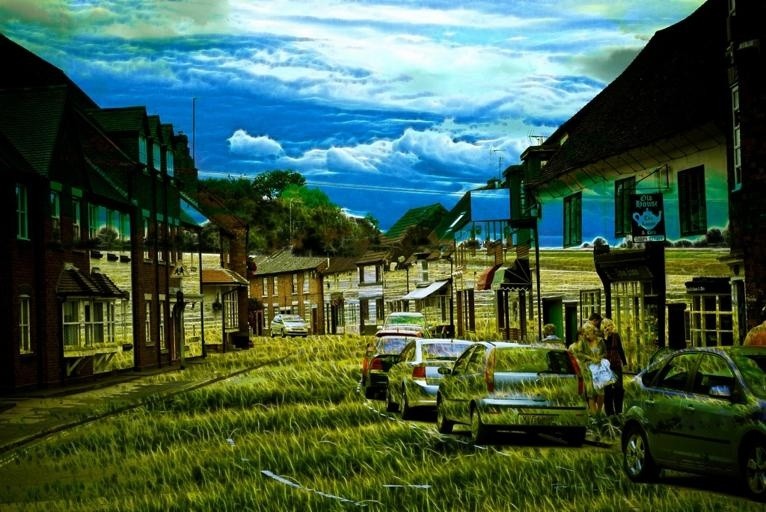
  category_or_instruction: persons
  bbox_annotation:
[540,313,631,433]
[742,306,766,347]
